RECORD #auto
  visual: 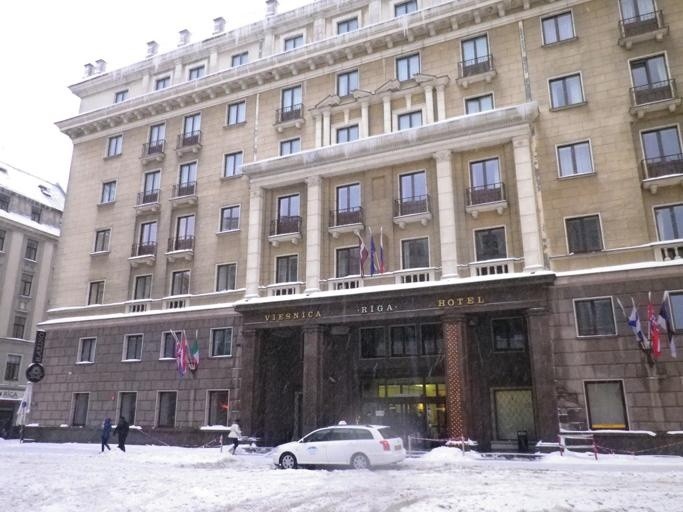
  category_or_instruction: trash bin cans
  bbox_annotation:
[517,431,529,451]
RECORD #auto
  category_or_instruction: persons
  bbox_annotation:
[358,410,373,425]
[227,419,243,455]
[98,417,112,454]
[112,416,130,452]
[417,411,426,434]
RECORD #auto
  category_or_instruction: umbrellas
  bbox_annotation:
[15,381,33,444]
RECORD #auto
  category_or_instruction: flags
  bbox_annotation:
[358,237,369,279]
[377,244,384,274]
[175,332,200,377]
[625,294,677,359]
[369,234,376,277]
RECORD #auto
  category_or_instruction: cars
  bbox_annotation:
[273,420,407,471]
